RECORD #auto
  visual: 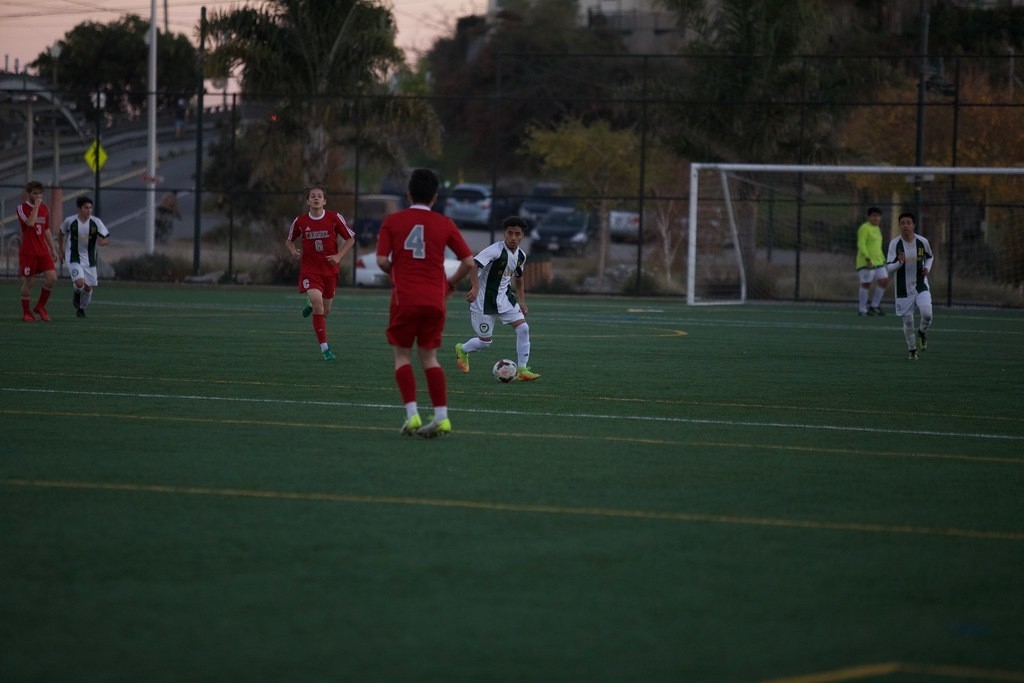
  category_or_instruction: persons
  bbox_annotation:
[856,206,889,316]
[59,196,110,319]
[376,169,474,438]
[16,180,58,322]
[154,189,182,242]
[885,212,935,361]
[173,97,190,139]
[285,185,355,362]
[455,218,540,382]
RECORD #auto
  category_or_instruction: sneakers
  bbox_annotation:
[34,304,51,322]
[23,313,37,322]
[77,308,86,319]
[321,347,336,360]
[916,328,928,351]
[909,350,919,361]
[303,295,314,318]
[417,416,451,438]
[399,414,423,437]
[456,342,469,373]
[73,291,81,310]
[517,366,542,380]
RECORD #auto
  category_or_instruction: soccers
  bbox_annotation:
[492,359,519,384]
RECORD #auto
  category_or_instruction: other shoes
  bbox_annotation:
[857,311,872,317]
[869,305,886,317]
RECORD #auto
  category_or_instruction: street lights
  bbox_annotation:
[10,92,37,182]
[46,42,65,239]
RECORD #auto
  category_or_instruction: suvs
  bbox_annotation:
[518,184,574,231]
[530,205,597,259]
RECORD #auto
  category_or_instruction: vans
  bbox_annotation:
[444,182,515,228]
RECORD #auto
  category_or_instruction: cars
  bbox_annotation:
[606,199,659,242]
[354,245,483,289]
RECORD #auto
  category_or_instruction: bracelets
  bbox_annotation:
[865,257,871,261]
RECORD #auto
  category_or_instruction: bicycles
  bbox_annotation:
[154,207,175,243]
